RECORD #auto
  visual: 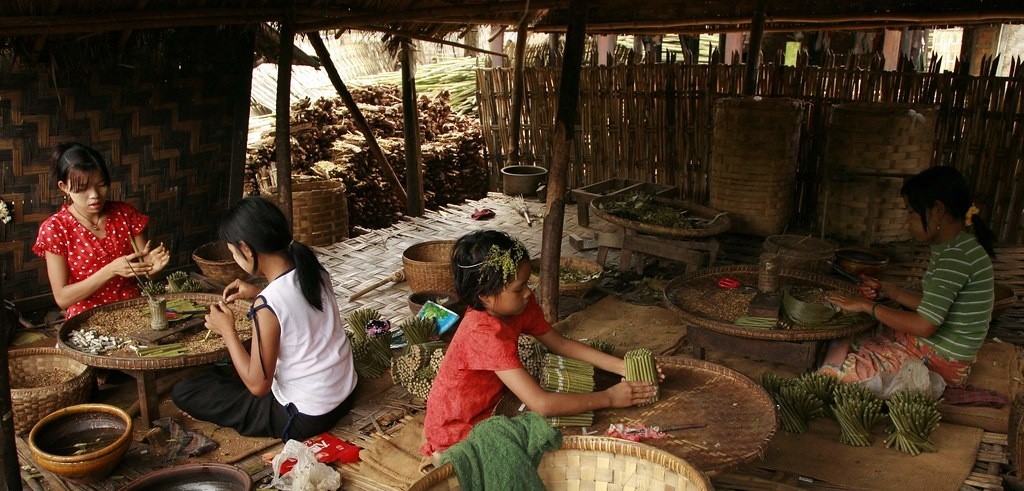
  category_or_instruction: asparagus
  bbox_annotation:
[752,370,946,457]
[342,308,451,399]
[136,270,211,360]
[735,300,864,330]
[596,190,707,229]
[514,332,658,428]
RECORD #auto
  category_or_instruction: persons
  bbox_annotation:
[171,196,358,442]
[813,163,997,388]
[32,140,171,392]
[419,229,665,473]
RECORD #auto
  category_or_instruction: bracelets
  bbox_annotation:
[871,301,884,321]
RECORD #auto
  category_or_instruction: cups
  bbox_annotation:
[146,298,169,331]
[757,254,781,294]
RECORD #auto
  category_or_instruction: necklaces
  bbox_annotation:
[71,203,103,231]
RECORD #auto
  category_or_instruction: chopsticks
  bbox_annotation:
[774,224,812,255]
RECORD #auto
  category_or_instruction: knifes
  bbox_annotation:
[626,423,707,434]
[177,310,208,314]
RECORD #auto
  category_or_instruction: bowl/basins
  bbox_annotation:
[27,404,133,486]
[407,290,459,316]
[116,463,254,491]
[835,247,890,273]
[500,165,549,197]
